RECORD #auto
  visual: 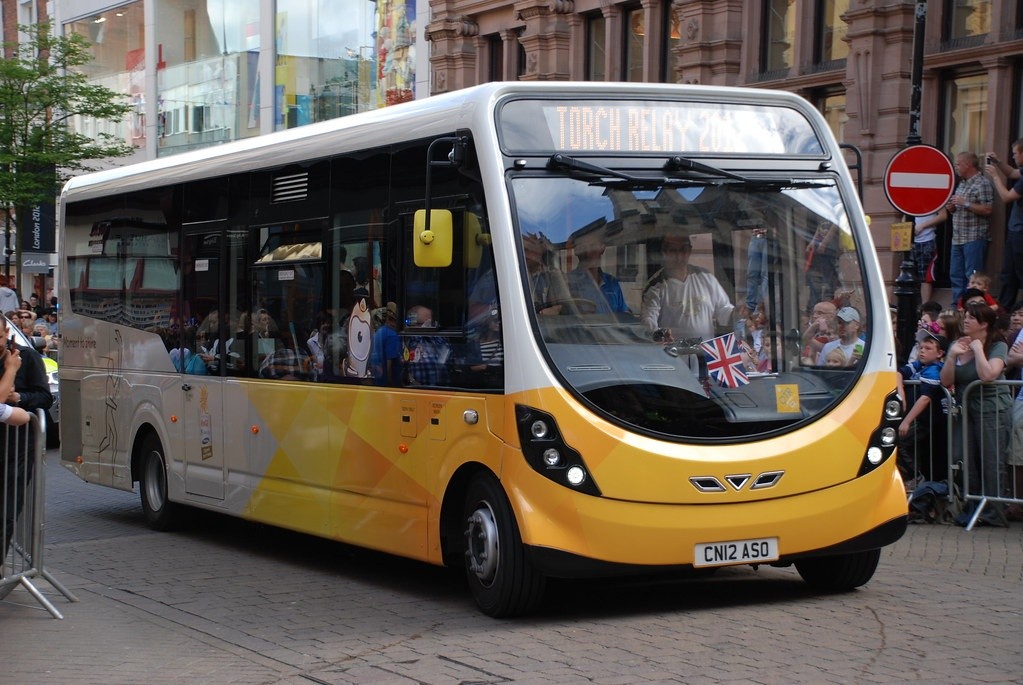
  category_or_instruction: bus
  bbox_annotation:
[56,77,908,615]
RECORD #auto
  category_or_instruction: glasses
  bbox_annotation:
[19,316,29,318]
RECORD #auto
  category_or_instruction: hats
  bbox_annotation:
[48,311,56,315]
[962,288,984,308]
[837,307,860,323]
[386,303,396,318]
[916,329,949,353]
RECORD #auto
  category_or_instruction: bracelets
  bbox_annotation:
[964,201,971,208]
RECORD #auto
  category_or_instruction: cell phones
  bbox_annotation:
[10,334,15,355]
[987,157,991,165]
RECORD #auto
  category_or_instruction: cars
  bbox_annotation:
[3,316,59,449]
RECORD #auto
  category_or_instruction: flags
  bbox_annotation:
[700,332,749,389]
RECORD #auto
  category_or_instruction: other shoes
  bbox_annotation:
[1005,506,1023,521]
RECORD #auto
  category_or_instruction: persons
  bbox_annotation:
[0,312,54,579]
[142,290,481,388]
[890,138,1023,526]
[800,192,864,369]
[468,226,571,330]
[0,402,30,426]
[5,293,60,362]
[559,230,633,316]
[738,226,785,373]
[642,226,748,377]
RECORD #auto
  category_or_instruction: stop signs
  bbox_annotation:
[884,143,955,217]
